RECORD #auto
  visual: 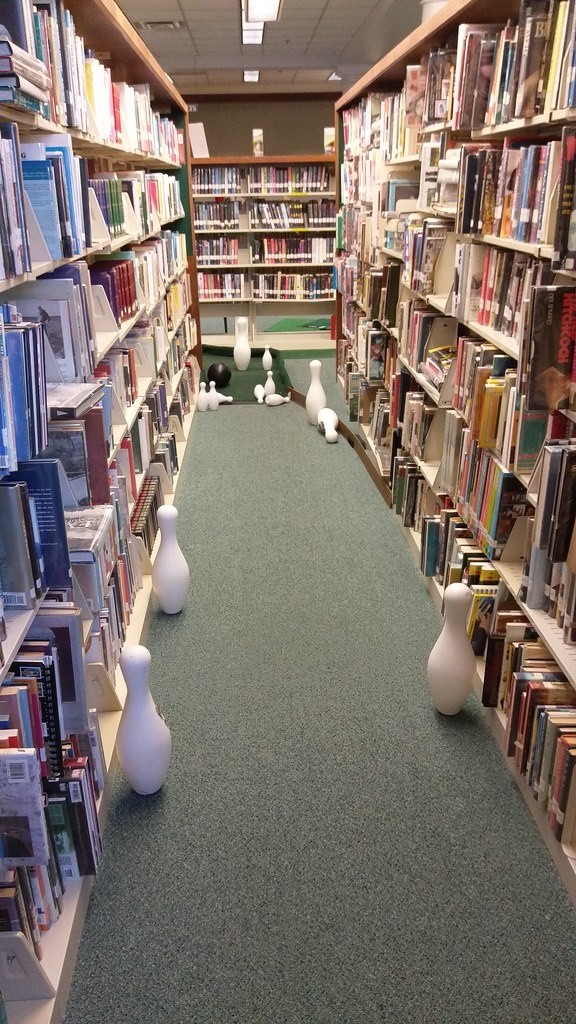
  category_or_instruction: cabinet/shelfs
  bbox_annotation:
[0,0,576,1024]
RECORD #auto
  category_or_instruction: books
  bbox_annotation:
[191,166,335,299]
[0,1,200,961]
[331,0,576,847]
